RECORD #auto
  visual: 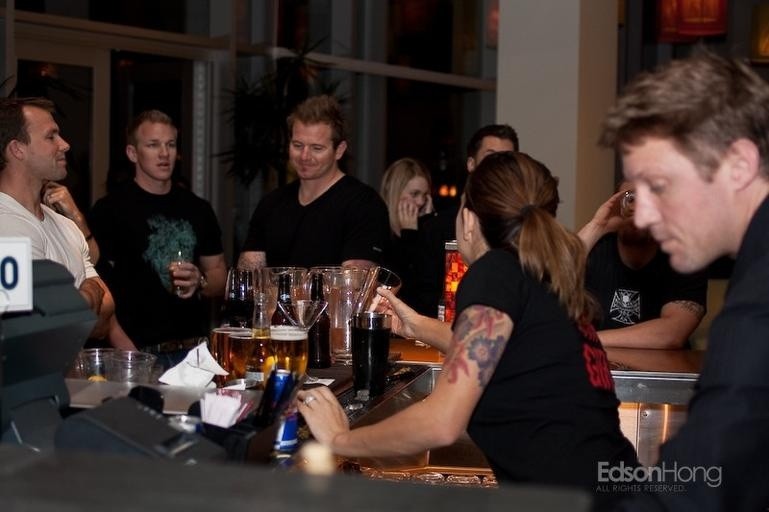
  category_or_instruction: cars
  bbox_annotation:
[73,345,157,384]
[345,264,402,325]
[209,326,309,391]
[620,191,637,217]
[352,314,391,397]
[169,249,188,295]
[353,465,500,488]
[325,270,367,365]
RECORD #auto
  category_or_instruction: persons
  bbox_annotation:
[235,52,769,512]
[0,90,117,338]
[42,182,100,267]
[88,109,229,361]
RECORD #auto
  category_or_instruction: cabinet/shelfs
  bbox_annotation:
[221,265,332,371]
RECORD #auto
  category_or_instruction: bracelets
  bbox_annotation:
[86,233,94,241]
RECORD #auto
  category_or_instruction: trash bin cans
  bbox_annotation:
[200,269,209,290]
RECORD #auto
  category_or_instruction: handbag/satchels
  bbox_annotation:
[86,233,94,241]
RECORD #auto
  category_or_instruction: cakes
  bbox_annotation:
[271,368,298,453]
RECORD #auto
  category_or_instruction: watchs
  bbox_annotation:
[200,269,209,290]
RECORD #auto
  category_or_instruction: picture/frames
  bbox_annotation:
[388,337,703,468]
[261,364,433,477]
